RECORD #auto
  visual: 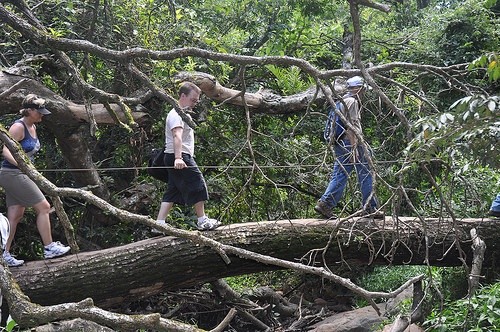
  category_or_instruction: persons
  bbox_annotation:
[487,192,500,216]
[0,94,71,267]
[314,75,384,216]
[151,81,220,235]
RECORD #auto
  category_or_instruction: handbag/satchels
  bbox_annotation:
[148,147,170,184]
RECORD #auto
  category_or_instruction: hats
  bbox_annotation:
[21,104,52,115]
[346,75,366,87]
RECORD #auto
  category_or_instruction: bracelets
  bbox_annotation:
[175,158,184,160]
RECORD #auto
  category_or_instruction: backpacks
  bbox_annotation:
[323,95,357,145]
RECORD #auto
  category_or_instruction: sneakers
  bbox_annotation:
[484,210,500,218]
[197,217,221,231]
[150,222,174,236]
[3,252,25,267]
[314,199,339,219]
[43,241,71,259]
[362,207,385,219]
